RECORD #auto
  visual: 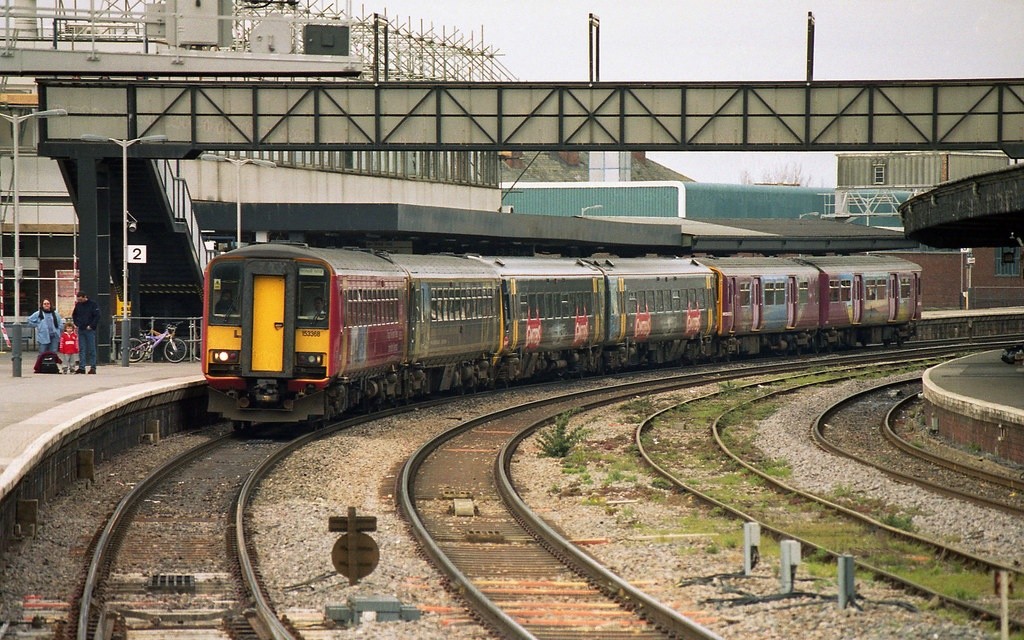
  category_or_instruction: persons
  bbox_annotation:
[215,289,237,314]
[306,297,325,315]
[28,298,64,373]
[59,322,79,373]
[72,291,100,374]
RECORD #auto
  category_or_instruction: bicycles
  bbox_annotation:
[189,324,201,362]
[119,321,188,363]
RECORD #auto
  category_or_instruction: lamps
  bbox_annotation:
[1001,246,1016,265]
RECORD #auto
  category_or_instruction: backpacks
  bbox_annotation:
[41,355,59,373]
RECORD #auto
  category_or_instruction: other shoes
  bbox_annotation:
[88,369,97,374]
[64,370,68,374]
[75,369,85,374]
[70,370,76,373]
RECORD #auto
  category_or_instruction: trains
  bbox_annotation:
[201,240,923,433]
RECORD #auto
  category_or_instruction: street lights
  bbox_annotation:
[0,109,68,377]
[581,205,602,216]
[201,154,276,248]
[81,134,168,367]
[799,212,819,219]
